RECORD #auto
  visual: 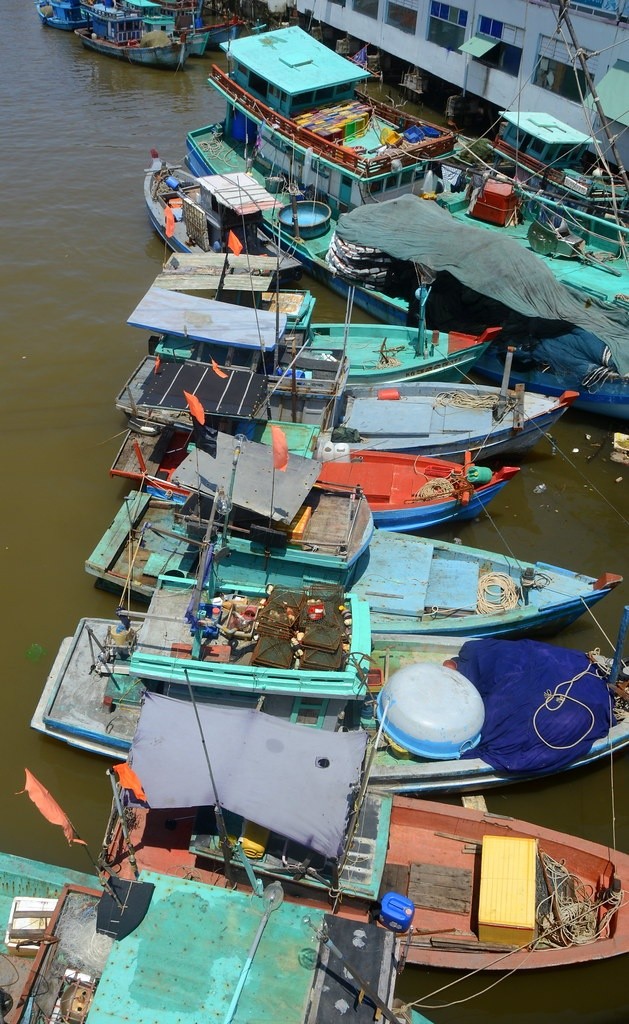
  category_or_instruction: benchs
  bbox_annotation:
[171,643,231,664]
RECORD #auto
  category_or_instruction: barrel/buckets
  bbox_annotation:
[377,389,400,399]
[323,441,351,462]
[378,891,415,934]
[467,466,492,484]
[166,176,180,189]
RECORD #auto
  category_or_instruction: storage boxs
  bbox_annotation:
[385,126,438,145]
[384,733,415,760]
[278,505,312,545]
[104,0,113,7]
[5,896,59,956]
[469,180,519,225]
[368,668,382,686]
[195,18,203,28]
[292,99,373,146]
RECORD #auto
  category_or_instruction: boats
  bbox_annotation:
[89,670,629,971]
[75,0,246,71]
[490,109,629,231]
[179,24,629,422]
[30,254,629,805]
[144,145,305,287]
[0,851,440,1024]
[34,0,95,31]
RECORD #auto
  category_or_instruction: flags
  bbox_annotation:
[212,360,229,378]
[164,206,174,238]
[184,390,205,425]
[272,426,288,472]
[227,229,243,256]
[113,763,151,809]
[14,768,87,846]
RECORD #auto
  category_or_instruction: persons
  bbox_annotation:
[40,2,54,18]
[125,37,140,48]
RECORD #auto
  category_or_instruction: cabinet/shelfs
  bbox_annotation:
[477,835,539,944]
